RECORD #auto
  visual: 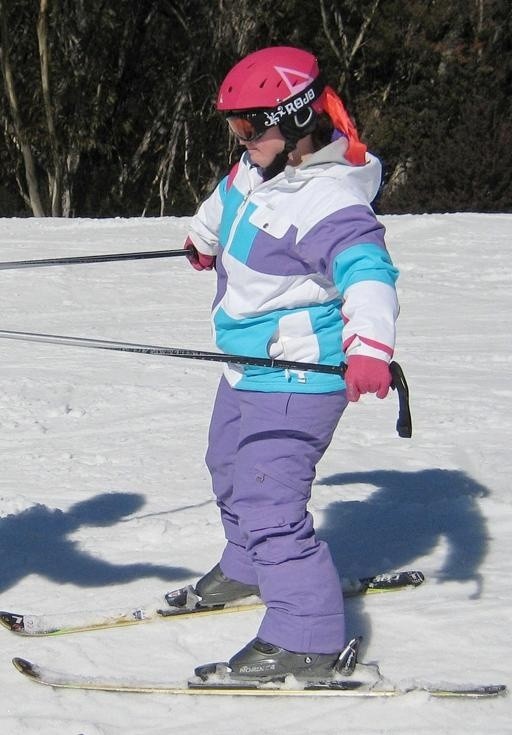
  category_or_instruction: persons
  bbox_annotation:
[165,46,400,685]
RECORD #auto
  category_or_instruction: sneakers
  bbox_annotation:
[195,562,261,607]
[228,636,341,681]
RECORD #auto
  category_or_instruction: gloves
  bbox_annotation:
[183,237,215,271]
[344,353,392,402]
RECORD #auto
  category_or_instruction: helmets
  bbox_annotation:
[214,46,321,113]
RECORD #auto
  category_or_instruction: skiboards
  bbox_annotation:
[1,573,506,698]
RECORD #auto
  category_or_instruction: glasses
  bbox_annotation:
[220,73,328,145]
